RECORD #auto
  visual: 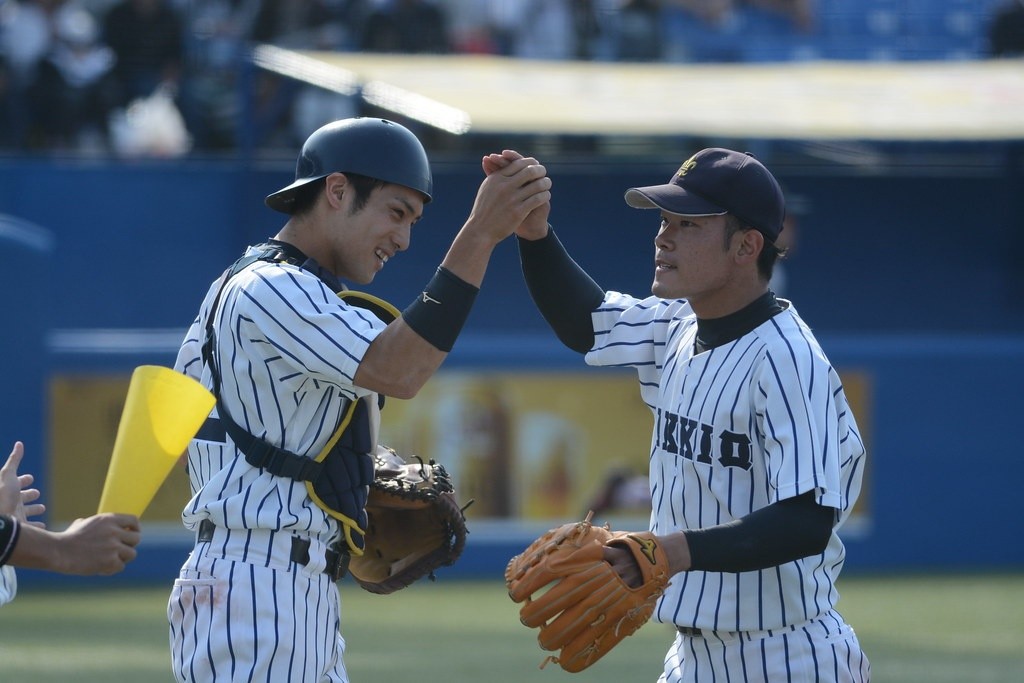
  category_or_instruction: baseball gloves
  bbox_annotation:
[346,443,469,595]
[504,511,672,675]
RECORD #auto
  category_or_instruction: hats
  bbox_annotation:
[624,148,785,241]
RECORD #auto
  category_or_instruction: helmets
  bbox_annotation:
[265,117,433,214]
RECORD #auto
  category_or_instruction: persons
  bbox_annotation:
[163,113,552,683]
[479,147,879,683]
[1,427,144,579]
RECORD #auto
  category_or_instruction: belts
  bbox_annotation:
[198,519,351,580]
[679,625,716,634]
